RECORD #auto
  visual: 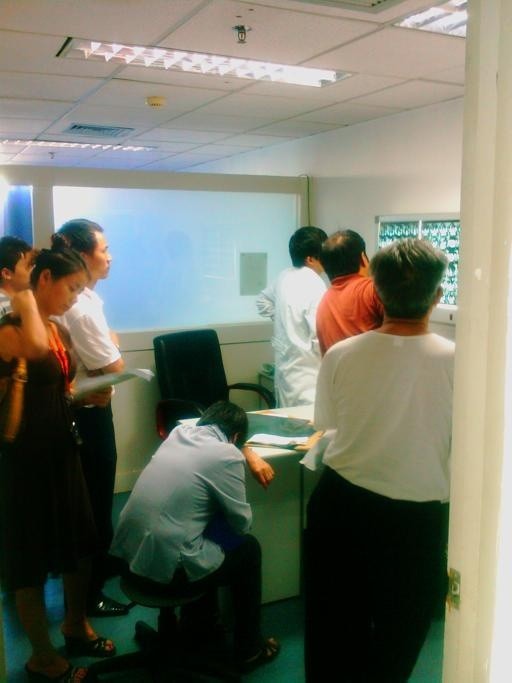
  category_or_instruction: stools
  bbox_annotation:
[86,561,220,682]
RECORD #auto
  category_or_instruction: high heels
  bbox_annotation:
[64,632,116,657]
[25,657,88,681]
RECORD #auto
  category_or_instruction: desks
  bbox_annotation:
[177,406,326,605]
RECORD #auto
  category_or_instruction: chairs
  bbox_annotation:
[151,327,274,439]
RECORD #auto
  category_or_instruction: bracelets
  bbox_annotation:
[13,284,32,290]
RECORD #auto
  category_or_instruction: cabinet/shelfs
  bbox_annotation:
[251,362,278,414]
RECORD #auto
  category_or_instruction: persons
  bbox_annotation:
[106,400,281,677]
[49,217,131,618]
[0,232,119,683]
[255,226,329,410]
[300,236,456,682]
[0,235,35,318]
[292,229,384,451]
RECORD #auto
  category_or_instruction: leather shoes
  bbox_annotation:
[88,598,128,617]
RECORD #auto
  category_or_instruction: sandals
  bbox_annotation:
[247,637,279,670]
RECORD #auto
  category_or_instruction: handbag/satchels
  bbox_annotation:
[1,373,25,440]
